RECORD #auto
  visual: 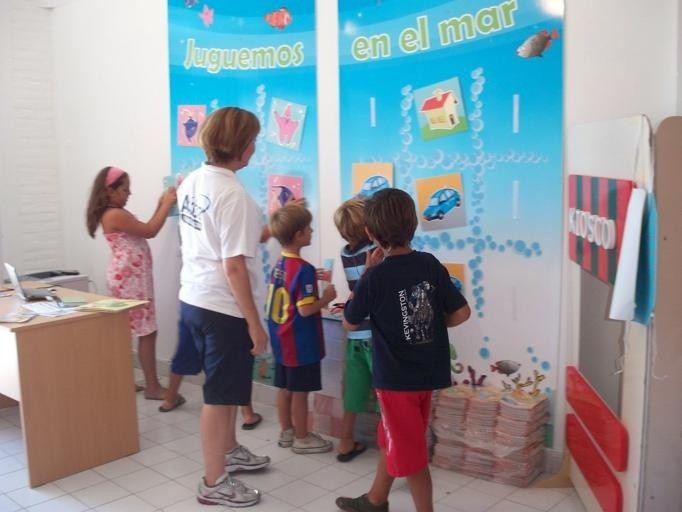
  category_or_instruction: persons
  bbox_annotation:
[335,198,376,461]
[85,166,176,401]
[269,205,335,454]
[160,314,263,430]
[173,107,268,509]
[335,188,471,511]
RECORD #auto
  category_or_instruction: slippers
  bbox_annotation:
[159,393,185,412]
[337,439,366,462]
[242,413,262,430]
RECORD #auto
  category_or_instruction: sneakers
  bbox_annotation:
[336,493,389,511]
[292,431,333,454]
[225,444,270,472]
[197,475,260,507]
[278,427,294,448]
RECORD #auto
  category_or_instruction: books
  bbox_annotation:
[0,295,149,322]
[305,382,549,488]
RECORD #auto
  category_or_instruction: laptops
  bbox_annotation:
[4,262,57,301]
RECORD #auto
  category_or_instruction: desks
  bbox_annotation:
[1,282,150,490]
